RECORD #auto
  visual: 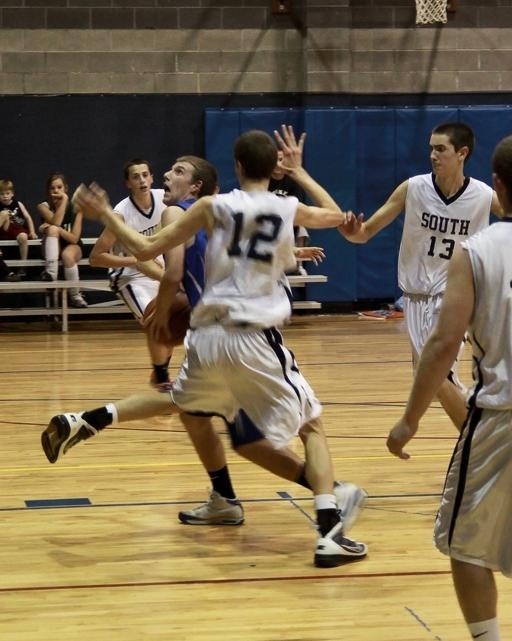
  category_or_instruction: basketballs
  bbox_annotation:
[144,291,193,347]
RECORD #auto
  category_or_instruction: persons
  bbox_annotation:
[268,146,310,277]
[142,154,367,531]
[41,124,369,568]
[0,255,21,282]
[0,179,38,277]
[386,136,511,640]
[90,158,173,393]
[339,122,505,434]
[37,171,88,308]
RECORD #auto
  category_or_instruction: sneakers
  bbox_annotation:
[334,482,369,534]
[314,520,367,568]
[70,292,87,307]
[150,371,175,393]
[296,266,307,275]
[41,411,99,465]
[41,267,56,292]
[178,490,244,526]
